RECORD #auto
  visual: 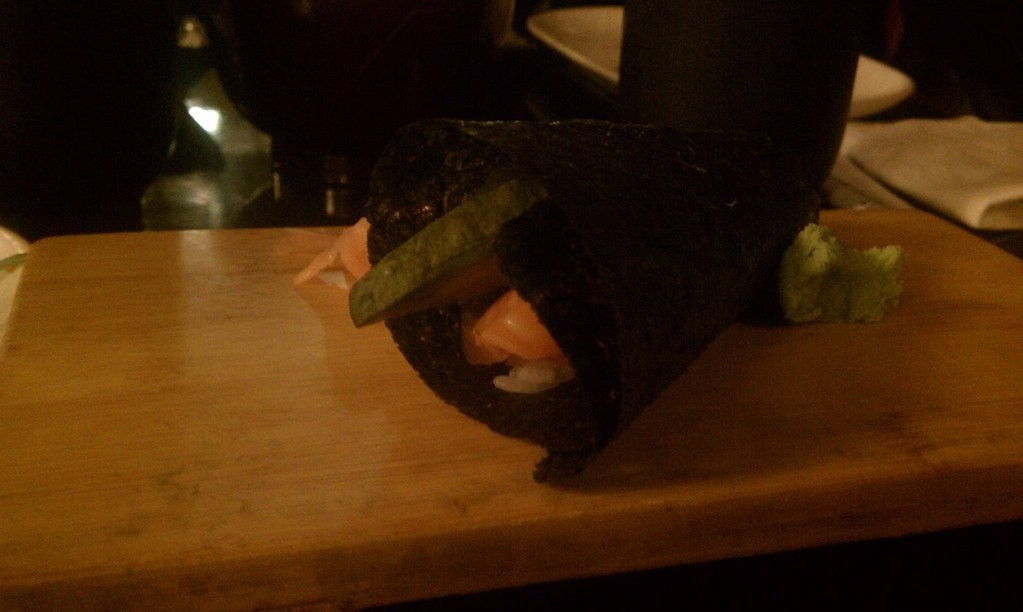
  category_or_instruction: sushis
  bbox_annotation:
[289,1,906,485]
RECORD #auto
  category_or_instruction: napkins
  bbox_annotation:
[845,116,1022,231]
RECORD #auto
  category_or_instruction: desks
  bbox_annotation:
[0,202,1019,611]
